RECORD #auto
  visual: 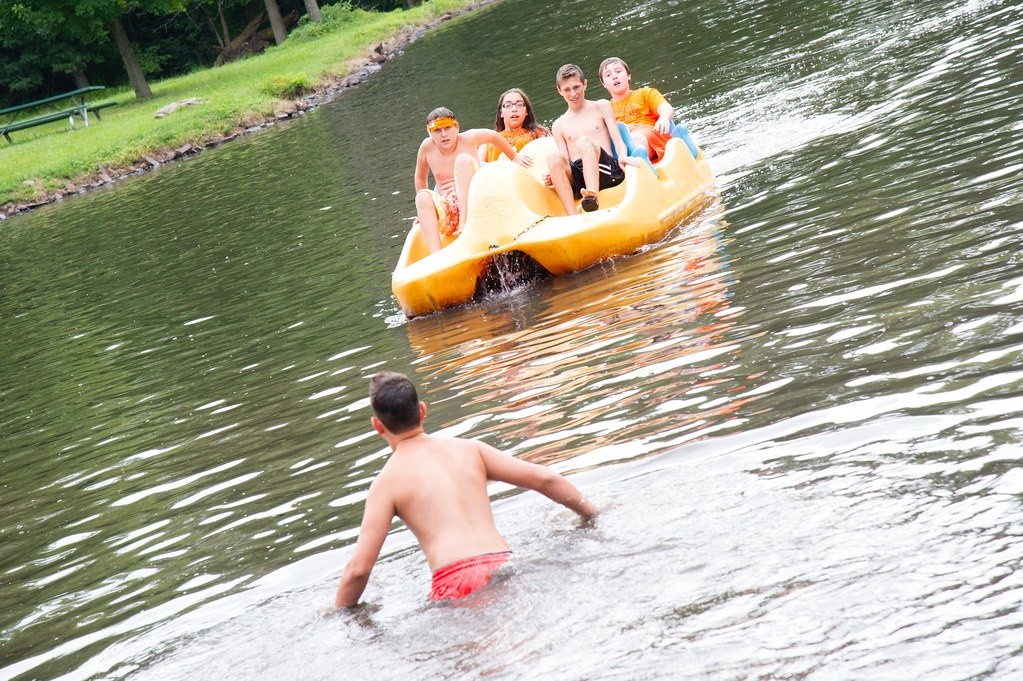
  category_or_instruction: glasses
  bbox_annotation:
[502,101,526,109]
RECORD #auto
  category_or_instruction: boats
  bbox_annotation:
[392,120,715,322]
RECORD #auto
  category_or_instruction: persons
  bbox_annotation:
[332,369,599,612]
[477,88,548,165]
[413,106,531,258]
[544,62,639,217]
[599,56,675,163]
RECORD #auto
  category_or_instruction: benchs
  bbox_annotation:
[69,100,120,129]
[0,101,88,143]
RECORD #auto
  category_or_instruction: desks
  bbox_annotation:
[0,83,107,146]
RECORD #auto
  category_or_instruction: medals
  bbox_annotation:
[511,146,518,152]
